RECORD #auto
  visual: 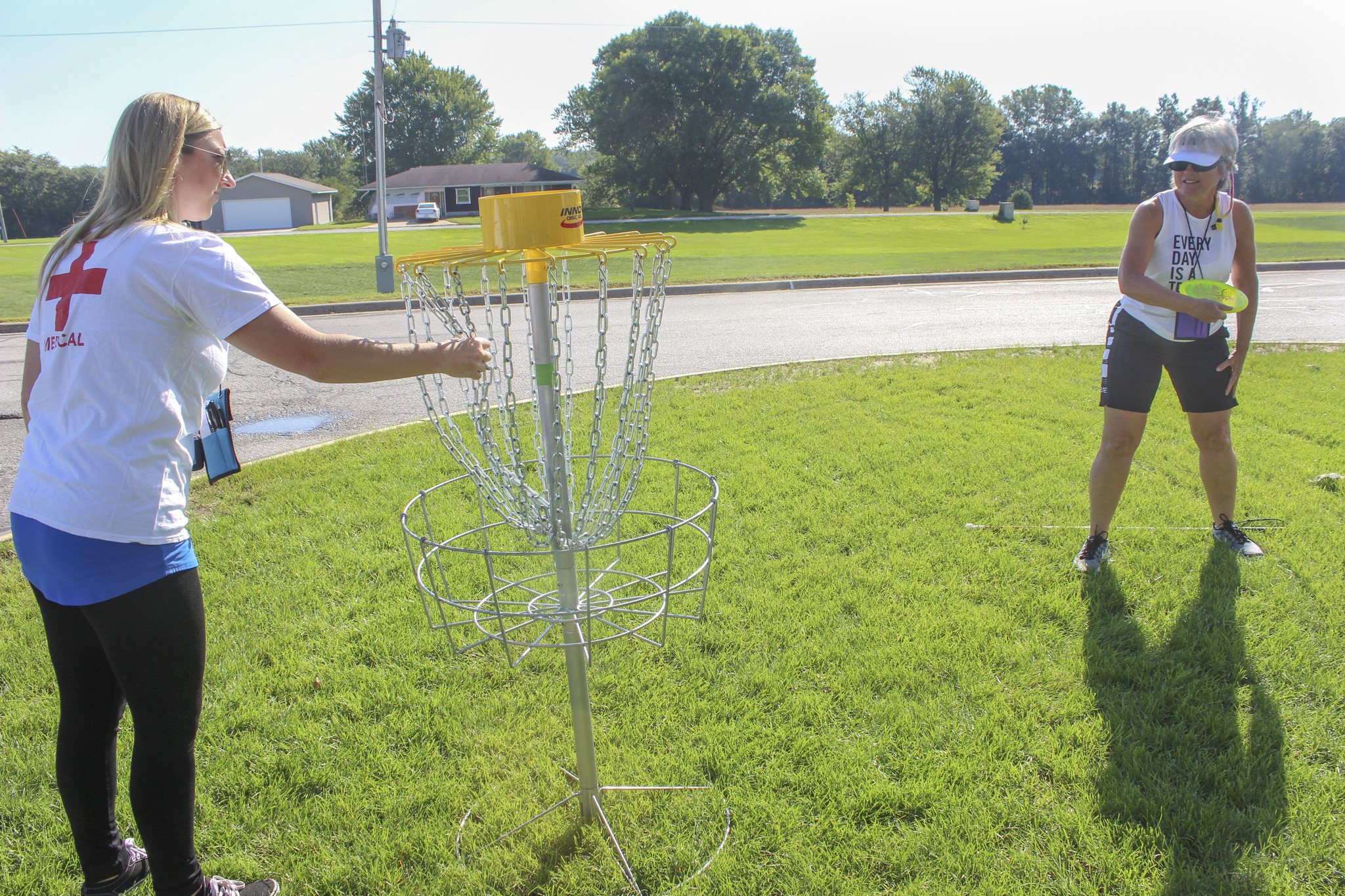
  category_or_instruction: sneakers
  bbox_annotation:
[194,875,281,896]
[80,838,151,896]
[1074,523,1110,574]
[1211,513,1264,559]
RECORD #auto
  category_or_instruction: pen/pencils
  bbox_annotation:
[205,400,225,433]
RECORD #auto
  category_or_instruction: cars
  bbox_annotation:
[415,202,440,222]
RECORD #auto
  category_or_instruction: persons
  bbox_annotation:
[1073,114,1263,574]
[9,93,493,896]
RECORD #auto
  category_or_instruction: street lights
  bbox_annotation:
[358,101,368,185]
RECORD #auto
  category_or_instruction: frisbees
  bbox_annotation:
[1179,279,1249,314]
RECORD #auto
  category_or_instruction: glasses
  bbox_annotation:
[184,143,230,178]
[1168,158,1225,172]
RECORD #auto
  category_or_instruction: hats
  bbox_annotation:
[1163,144,1222,167]
